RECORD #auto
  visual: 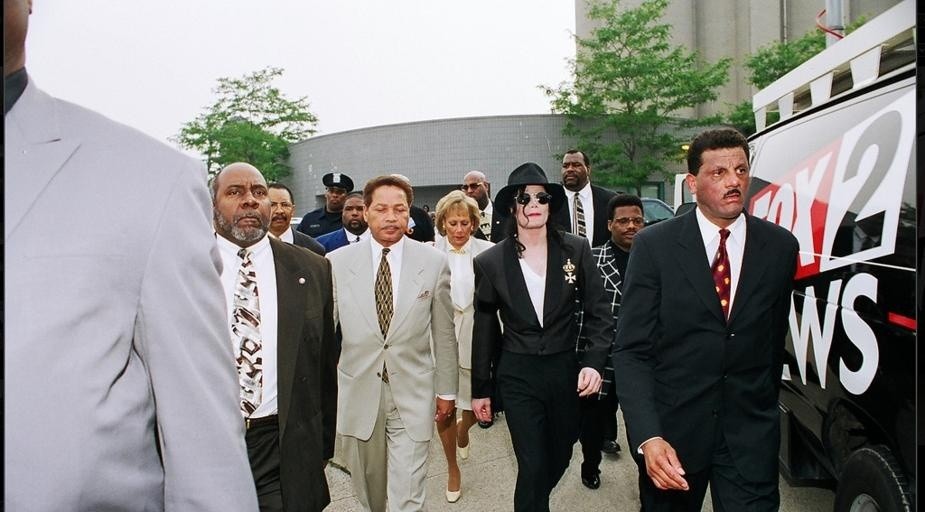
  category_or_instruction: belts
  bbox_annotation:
[245,415,278,429]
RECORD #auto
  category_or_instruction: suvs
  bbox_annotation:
[671,0,925,512]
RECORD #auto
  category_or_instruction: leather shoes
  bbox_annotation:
[457,418,470,459]
[446,471,462,502]
[478,413,493,428]
[602,440,620,453]
[582,473,600,489]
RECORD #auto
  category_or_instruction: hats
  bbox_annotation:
[323,173,354,192]
[495,163,565,217]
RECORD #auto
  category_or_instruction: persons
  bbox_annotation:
[213,163,337,512]
[616,128,799,512]
[2,0,258,512]
[267,152,645,512]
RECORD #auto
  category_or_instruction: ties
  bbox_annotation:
[480,212,491,240]
[711,228,730,321]
[375,248,393,384]
[230,248,262,418]
[575,193,586,237]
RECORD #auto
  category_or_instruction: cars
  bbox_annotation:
[636,194,677,224]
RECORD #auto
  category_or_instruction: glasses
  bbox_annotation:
[514,192,552,204]
[461,183,482,192]
[619,218,644,226]
